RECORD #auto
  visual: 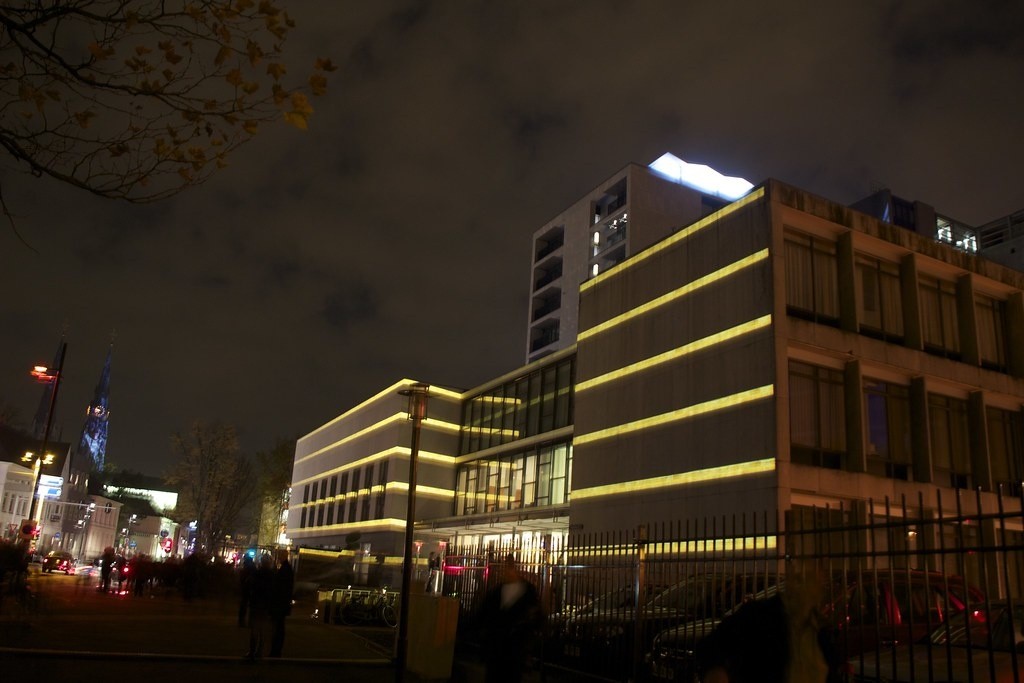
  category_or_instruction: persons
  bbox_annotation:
[96,546,297,660]
[426,543,850,683]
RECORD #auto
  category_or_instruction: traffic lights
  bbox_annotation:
[164,538,172,552]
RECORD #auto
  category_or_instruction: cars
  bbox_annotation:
[42,551,77,576]
[547,573,1023,683]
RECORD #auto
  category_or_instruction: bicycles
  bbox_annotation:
[339,591,399,626]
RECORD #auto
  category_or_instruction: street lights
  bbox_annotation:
[9,366,62,589]
